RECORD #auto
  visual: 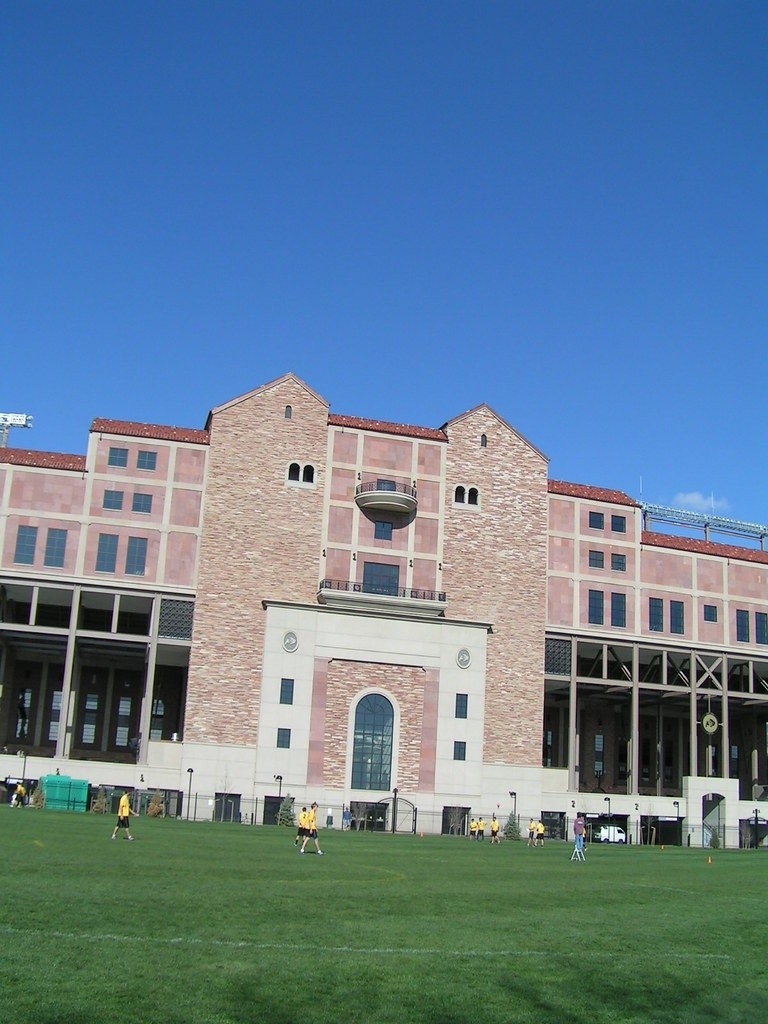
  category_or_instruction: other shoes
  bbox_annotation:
[293,842,297,847]
[128,836,134,841]
[299,850,304,855]
[317,851,324,855]
[112,835,116,839]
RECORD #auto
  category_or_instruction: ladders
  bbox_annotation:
[570,844,586,861]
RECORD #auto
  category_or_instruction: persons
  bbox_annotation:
[525,818,536,847]
[15,783,24,807]
[343,807,352,832]
[111,789,138,841]
[533,820,545,848]
[294,807,309,847]
[582,826,588,851]
[469,819,477,840]
[490,817,501,844]
[300,801,327,856]
[477,817,487,840]
[574,812,585,851]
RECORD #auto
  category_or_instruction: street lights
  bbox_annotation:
[186,769,195,818]
[603,797,611,845]
[673,800,682,846]
[16,749,27,782]
[509,791,518,834]
[272,774,283,824]
[392,787,399,834]
